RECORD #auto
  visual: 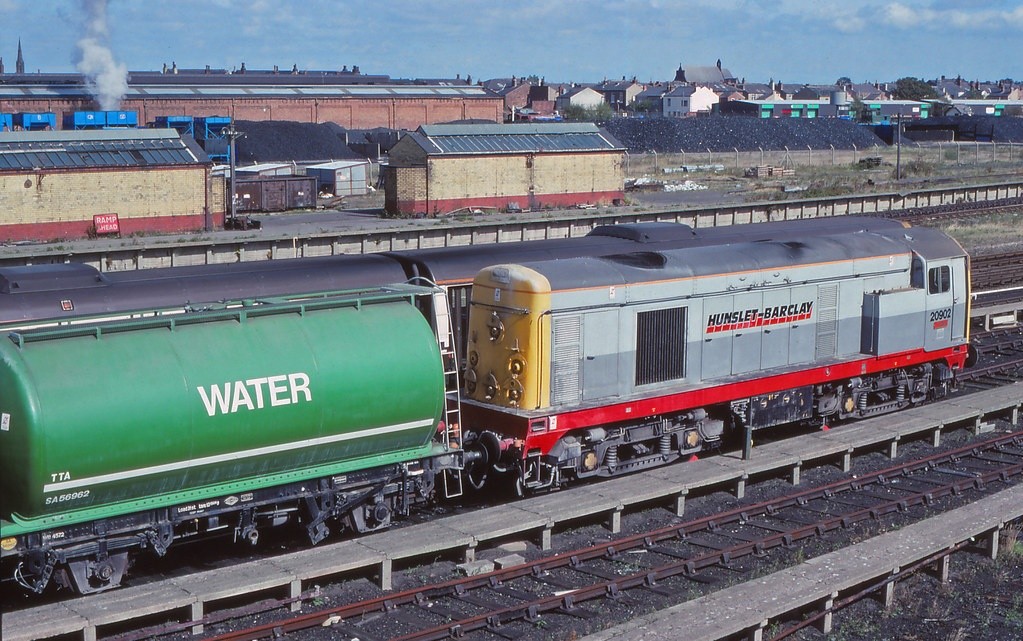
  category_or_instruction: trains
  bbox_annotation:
[0,212,978,597]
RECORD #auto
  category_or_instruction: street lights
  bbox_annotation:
[896,113,905,180]
[221,125,238,217]
